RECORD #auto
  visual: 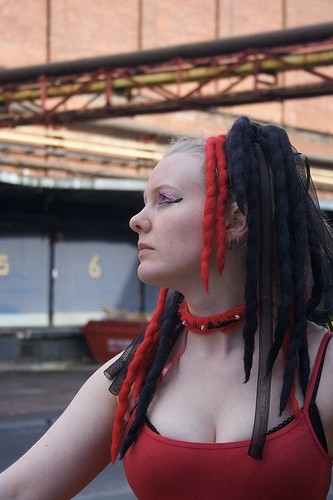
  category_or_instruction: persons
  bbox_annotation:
[0,116,331,500]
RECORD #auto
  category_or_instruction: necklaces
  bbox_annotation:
[174,295,246,338]
